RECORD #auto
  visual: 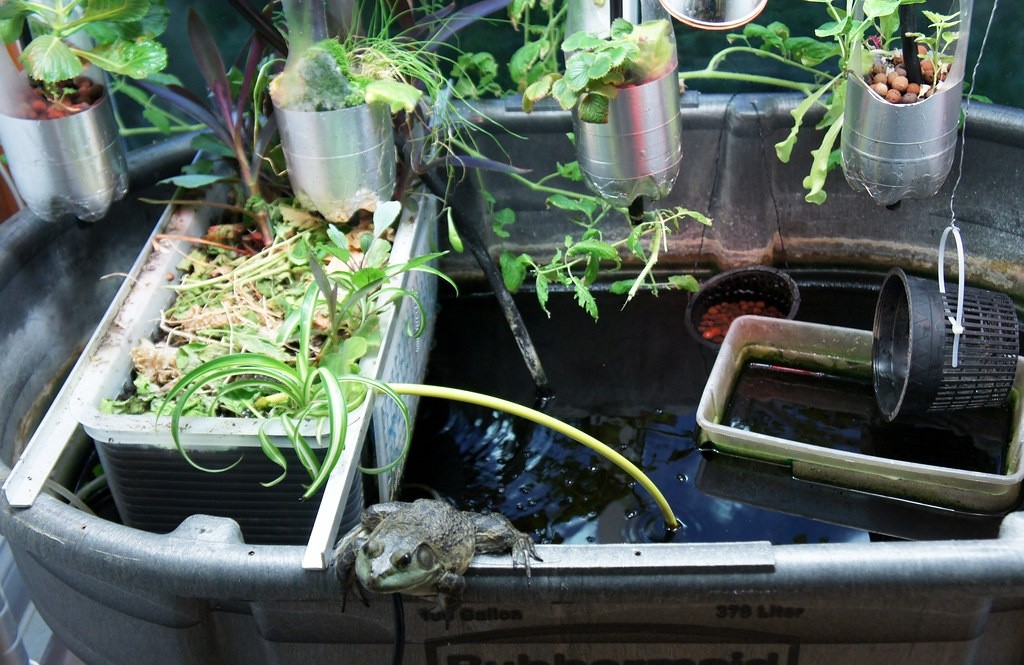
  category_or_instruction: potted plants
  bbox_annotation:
[0,0,958,545]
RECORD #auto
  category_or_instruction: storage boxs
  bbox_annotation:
[692,312,1024,509]
[691,424,1024,540]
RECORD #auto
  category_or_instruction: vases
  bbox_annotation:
[870,267,1018,423]
